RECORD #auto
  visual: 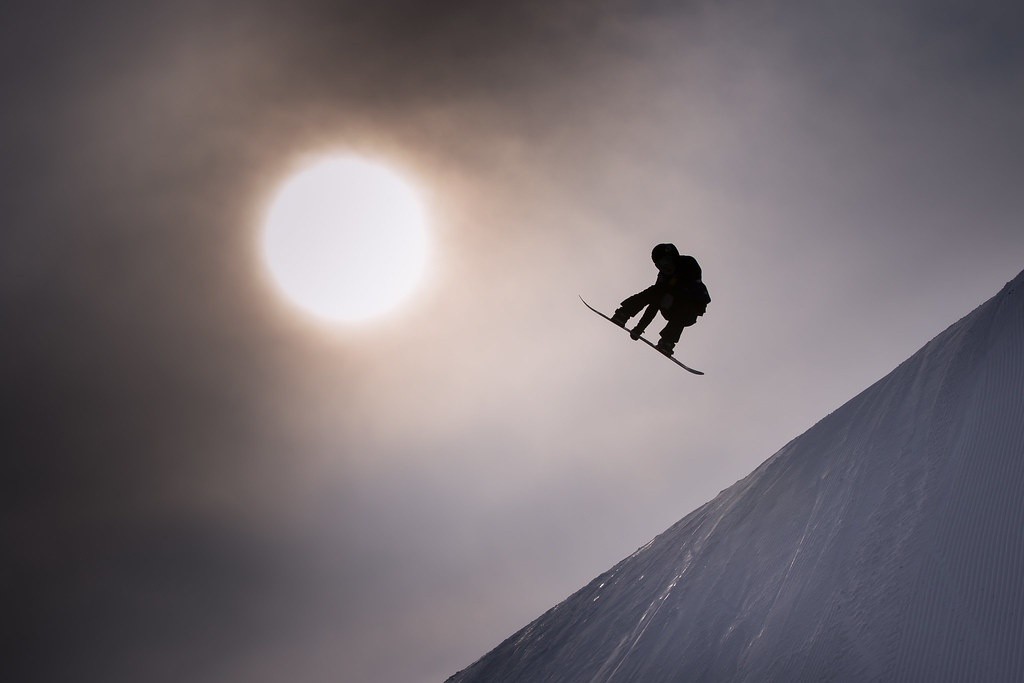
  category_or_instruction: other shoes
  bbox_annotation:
[655,337,677,355]
[611,306,631,326]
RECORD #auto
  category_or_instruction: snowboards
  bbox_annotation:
[579,295,705,375]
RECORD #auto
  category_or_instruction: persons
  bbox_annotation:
[612,243,711,355]
